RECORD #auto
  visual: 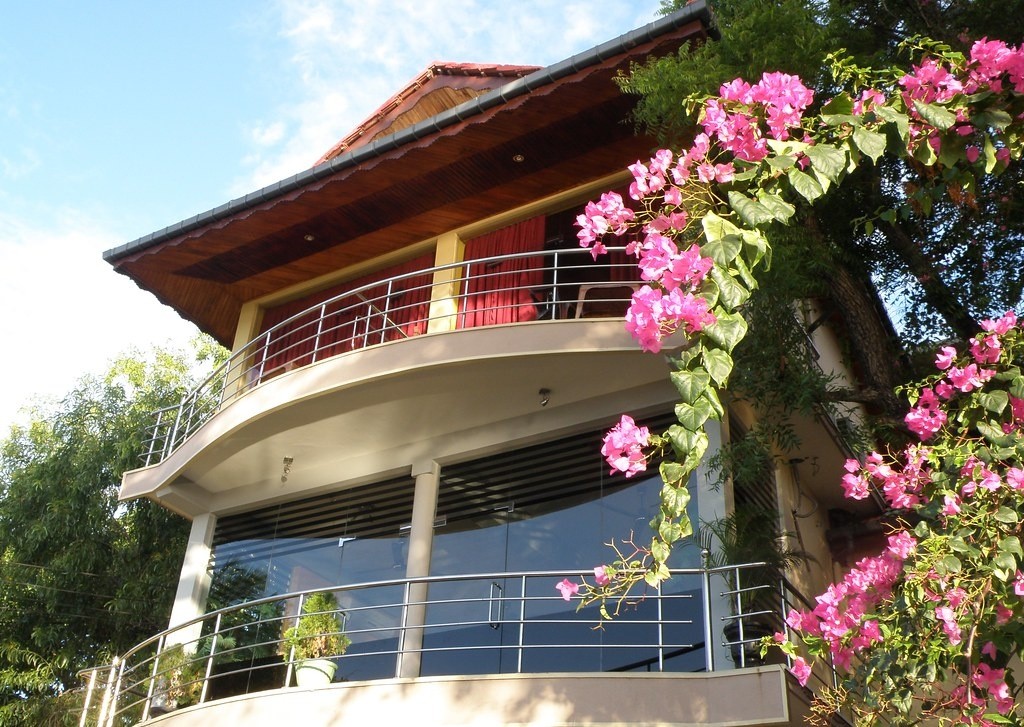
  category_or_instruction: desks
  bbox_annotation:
[574,283,640,318]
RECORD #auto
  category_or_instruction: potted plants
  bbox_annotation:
[673,507,825,667]
[276,591,353,686]
[142,643,186,713]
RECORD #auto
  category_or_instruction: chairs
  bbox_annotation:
[530,287,572,319]
